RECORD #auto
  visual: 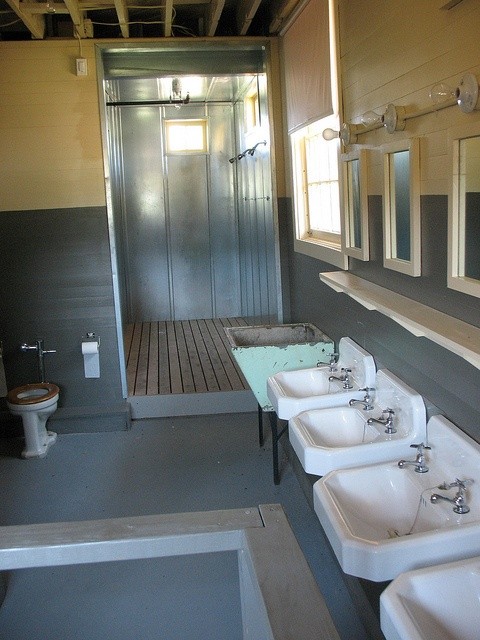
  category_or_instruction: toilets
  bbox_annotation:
[6,383,59,458]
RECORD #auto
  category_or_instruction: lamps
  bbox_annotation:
[359,104,398,134]
[431,73,477,114]
[324,122,352,148]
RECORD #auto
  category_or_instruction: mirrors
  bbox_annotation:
[340,147,369,262]
[379,138,423,279]
[446,123,480,302]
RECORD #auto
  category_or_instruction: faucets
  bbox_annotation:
[317,352,338,371]
[398,442,431,473]
[349,387,375,410]
[367,409,397,434]
[329,369,352,388]
[430,479,470,514]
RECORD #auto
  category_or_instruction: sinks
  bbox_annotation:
[314,415,480,582]
[267,336,376,420]
[380,557,480,640]
[288,370,427,477]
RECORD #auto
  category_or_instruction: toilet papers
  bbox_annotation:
[82,342,100,379]
